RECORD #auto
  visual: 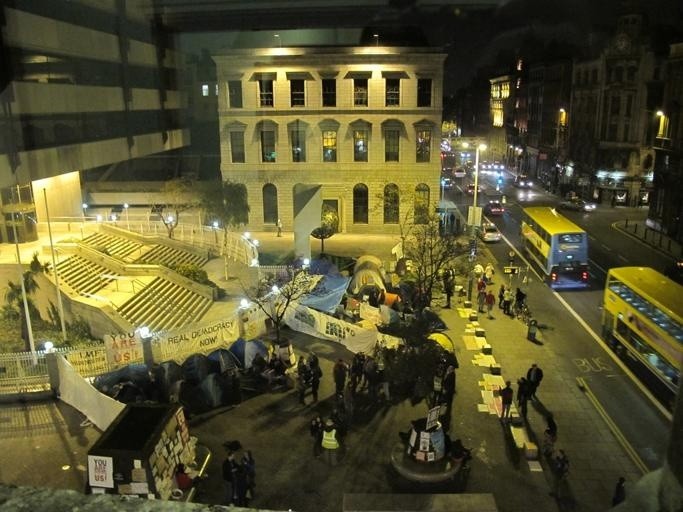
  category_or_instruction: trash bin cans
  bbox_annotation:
[528,319,537,340]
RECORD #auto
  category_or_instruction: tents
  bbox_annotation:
[350,254,385,295]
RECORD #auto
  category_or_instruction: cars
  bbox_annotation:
[559,197,595,212]
[442,151,504,189]
[481,223,501,242]
[484,201,504,216]
[515,175,529,188]
[466,184,480,195]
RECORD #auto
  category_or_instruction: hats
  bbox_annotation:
[325,418,335,426]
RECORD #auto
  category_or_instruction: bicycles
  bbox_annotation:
[517,307,529,325]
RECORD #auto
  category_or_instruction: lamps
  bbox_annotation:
[303,258,310,266]
[243,231,260,266]
[141,327,150,336]
[272,285,278,292]
[240,299,248,308]
[214,222,219,227]
[44,341,53,350]
[168,217,174,222]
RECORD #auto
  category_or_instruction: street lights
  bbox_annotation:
[462,142,486,236]
[124,203,129,230]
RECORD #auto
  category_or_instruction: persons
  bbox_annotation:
[497,380,513,423]
[371,292,378,308]
[473,261,526,320]
[220,451,237,506]
[295,343,406,468]
[243,448,255,501]
[543,413,557,444]
[526,362,543,400]
[368,285,378,306]
[275,217,282,237]
[235,455,248,507]
[548,449,570,499]
[613,475,626,506]
[515,375,528,421]
[376,288,385,308]
[175,462,192,489]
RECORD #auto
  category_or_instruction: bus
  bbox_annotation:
[520,207,589,289]
[602,266,683,422]
